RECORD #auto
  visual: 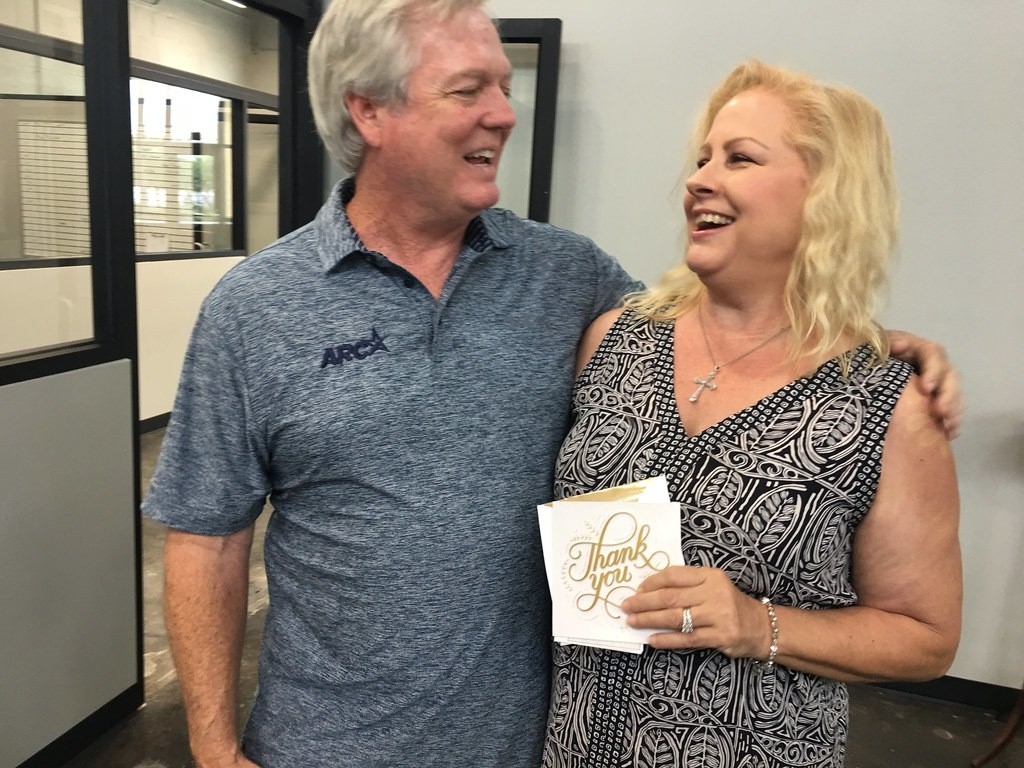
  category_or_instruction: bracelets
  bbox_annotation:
[750,596,778,672]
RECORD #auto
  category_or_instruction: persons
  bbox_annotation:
[139,0,965,768]
[540,62,965,768]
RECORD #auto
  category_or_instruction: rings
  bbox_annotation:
[681,608,695,633]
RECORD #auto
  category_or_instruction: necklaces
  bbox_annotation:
[690,295,792,403]
